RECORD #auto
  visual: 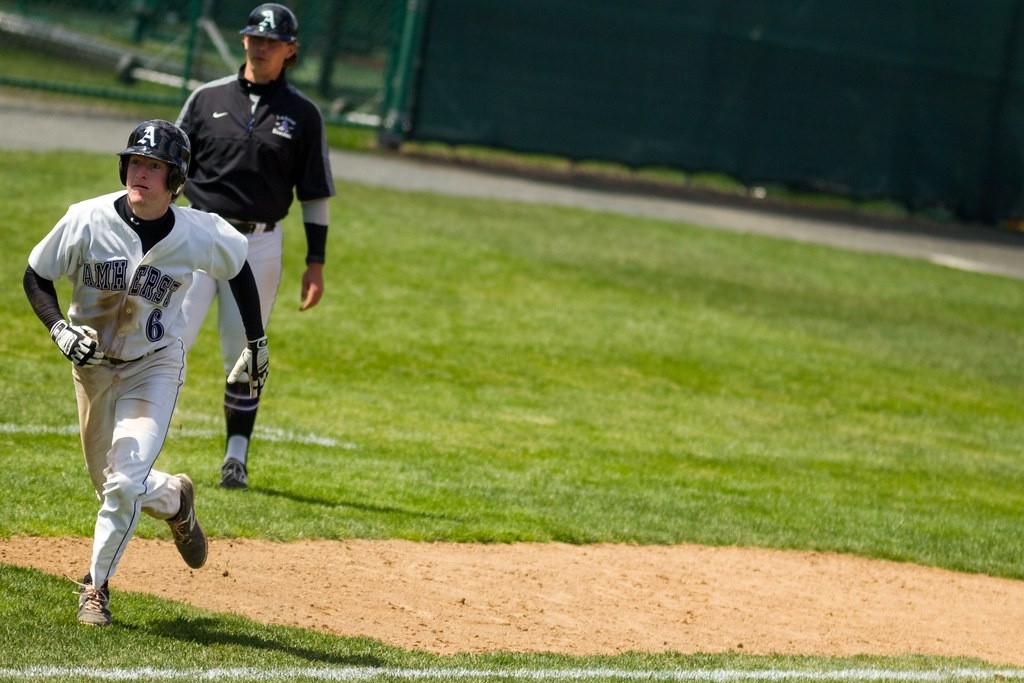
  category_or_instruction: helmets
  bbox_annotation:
[116,120,191,195]
[239,4,299,42]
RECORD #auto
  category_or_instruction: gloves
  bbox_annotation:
[50,320,104,369]
[228,335,270,399]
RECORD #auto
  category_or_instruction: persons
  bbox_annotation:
[26,120,270,627]
[172,3,334,486]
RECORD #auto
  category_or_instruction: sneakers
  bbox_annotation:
[63,573,110,626]
[164,474,207,568]
[220,458,248,490]
